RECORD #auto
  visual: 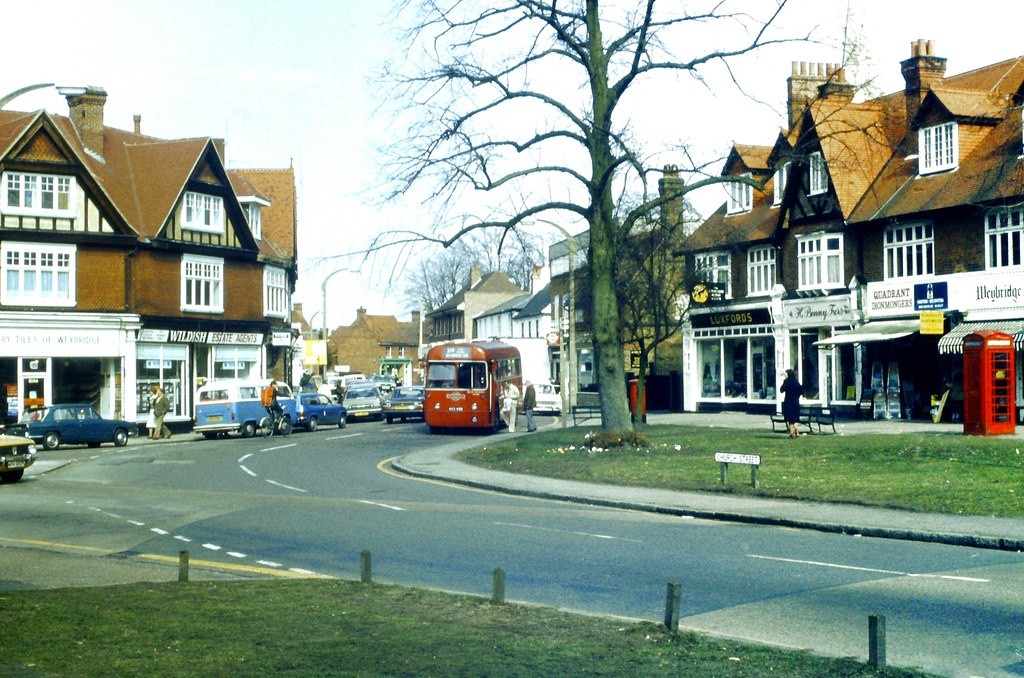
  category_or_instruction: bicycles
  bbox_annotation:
[259,406,293,440]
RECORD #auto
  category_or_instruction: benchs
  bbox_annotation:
[572,405,602,426]
[771,406,838,435]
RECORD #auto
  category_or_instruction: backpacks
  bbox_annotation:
[261,387,274,406]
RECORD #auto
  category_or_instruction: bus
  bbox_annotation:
[424,338,523,434]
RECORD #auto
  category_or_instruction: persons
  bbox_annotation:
[500,380,521,432]
[523,380,537,431]
[780,370,804,440]
[264,380,291,424]
[146,387,173,439]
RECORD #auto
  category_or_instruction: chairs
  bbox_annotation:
[400,394,407,397]
[366,393,374,397]
[352,394,359,398]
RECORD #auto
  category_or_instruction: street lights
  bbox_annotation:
[322,266,360,382]
[309,311,323,374]
[411,298,422,387]
[520,218,567,428]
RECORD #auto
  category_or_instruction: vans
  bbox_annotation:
[192,379,299,440]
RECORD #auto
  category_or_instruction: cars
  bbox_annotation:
[337,388,385,423]
[291,394,348,432]
[382,386,425,425]
[0,403,137,451]
[313,372,397,398]
[523,380,562,415]
[0,434,37,482]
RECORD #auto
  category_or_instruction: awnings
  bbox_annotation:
[812,318,948,350]
[937,320,1024,355]
